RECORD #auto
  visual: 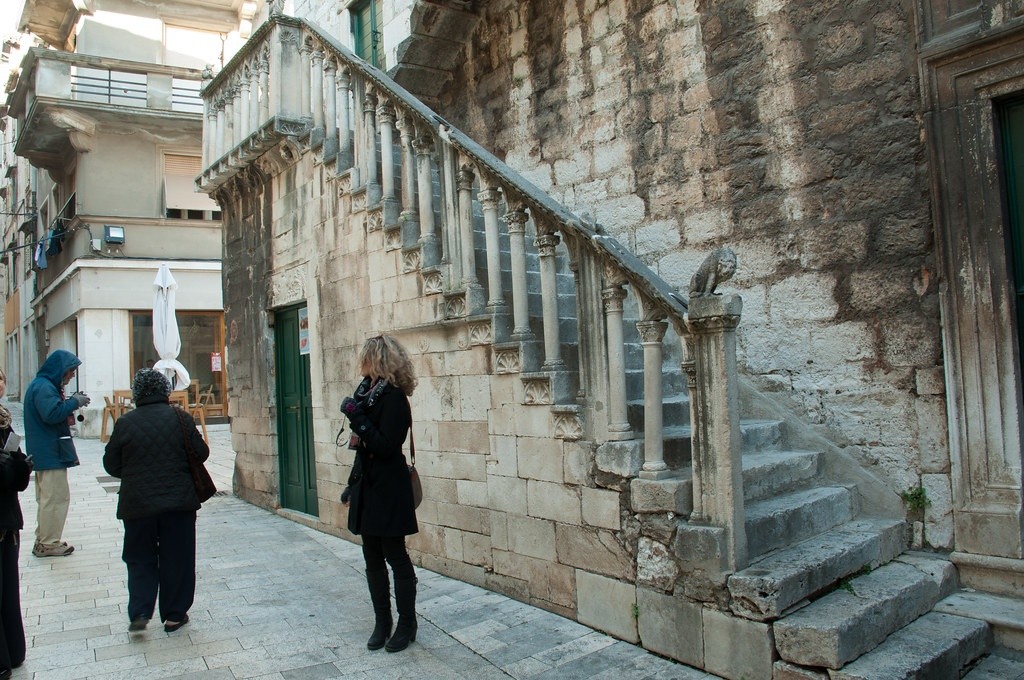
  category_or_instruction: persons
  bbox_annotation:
[103,367,211,634]
[0,369,34,680]
[22,348,90,558]
[339,330,420,654]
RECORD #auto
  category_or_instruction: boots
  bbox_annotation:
[366,556,418,651]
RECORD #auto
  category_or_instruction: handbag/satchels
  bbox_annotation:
[406,465,423,509]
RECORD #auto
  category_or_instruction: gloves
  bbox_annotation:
[72,391,91,407]
[341,398,358,414]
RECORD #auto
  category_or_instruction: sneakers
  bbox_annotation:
[33,540,73,557]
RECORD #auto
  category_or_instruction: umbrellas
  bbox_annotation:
[149,262,192,391]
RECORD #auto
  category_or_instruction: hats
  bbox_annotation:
[133,369,170,400]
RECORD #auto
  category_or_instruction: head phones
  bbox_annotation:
[336,427,351,447]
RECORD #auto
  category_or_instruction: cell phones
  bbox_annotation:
[24,454,33,462]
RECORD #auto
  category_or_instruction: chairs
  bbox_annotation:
[101,377,216,446]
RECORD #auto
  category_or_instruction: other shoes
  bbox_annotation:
[128,615,189,637]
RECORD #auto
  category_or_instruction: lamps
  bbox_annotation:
[104,224,126,255]
